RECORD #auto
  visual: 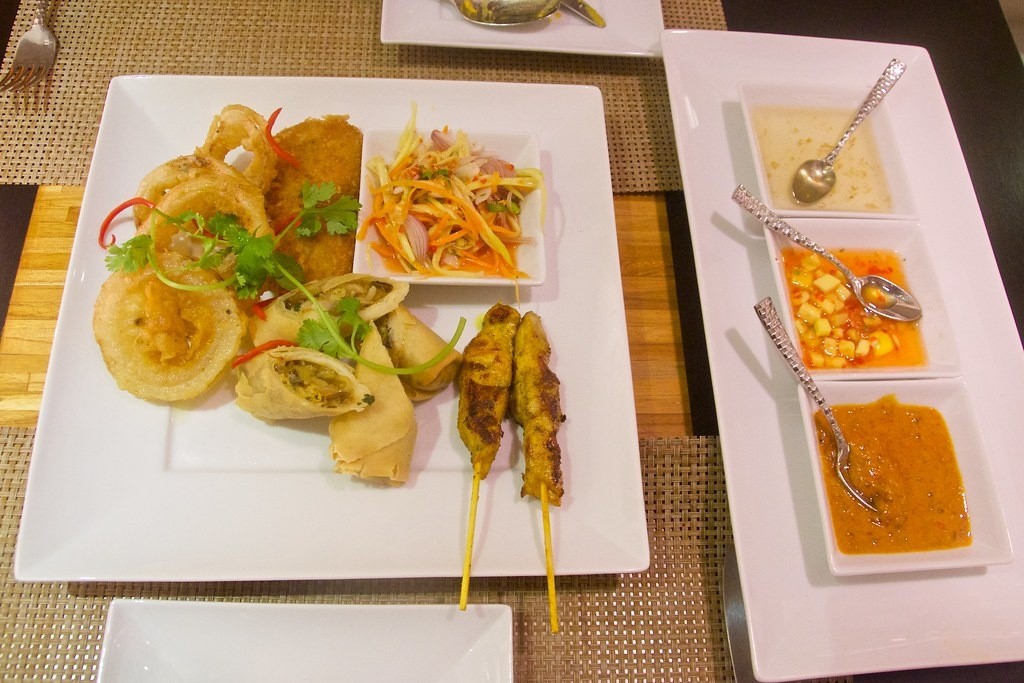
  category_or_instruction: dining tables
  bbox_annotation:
[2,0,1024,683]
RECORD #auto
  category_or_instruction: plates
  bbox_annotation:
[15,74,649,582]
[96,597,514,681]
[659,27,1023,682]
[766,218,964,379]
[797,379,1015,577]
[736,79,920,220]
[380,0,665,59]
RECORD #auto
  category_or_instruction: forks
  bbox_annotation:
[0,0,62,93]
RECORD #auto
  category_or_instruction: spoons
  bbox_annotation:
[731,184,922,321]
[792,58,908,202]
[754,297,881,513]
[451,0,561,27]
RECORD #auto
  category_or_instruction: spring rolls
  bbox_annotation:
[238,272,465,487]
